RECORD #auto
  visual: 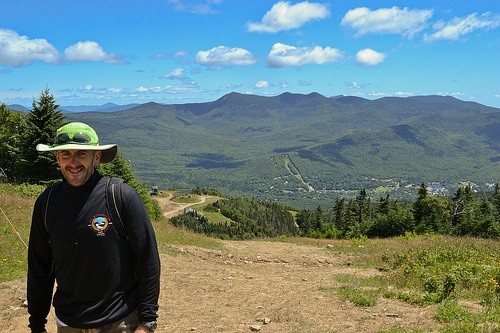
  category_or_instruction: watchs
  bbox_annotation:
[138,320,158,331]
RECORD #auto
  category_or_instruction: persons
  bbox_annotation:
[26,121,161,333]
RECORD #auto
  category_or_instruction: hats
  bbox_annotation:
[35,122,118,165]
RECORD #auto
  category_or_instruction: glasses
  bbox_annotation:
[54,132,91,144]
